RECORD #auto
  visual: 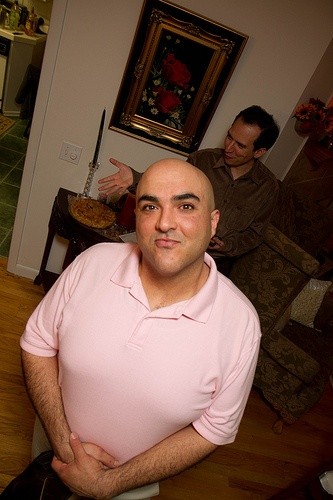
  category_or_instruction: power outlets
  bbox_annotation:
[58,140,83,165]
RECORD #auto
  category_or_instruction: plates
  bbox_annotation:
[39,24,49,34]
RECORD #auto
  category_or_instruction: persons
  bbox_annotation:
[97,105,280,277]
[20,158,261,500]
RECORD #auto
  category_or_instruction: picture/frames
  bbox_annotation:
[108,1,249,158]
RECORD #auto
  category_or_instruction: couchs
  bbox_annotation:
[223,222,333,434]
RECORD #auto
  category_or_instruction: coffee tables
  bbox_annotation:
[33,187,225,295]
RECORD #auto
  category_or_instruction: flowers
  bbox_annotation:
[292,98,327,120]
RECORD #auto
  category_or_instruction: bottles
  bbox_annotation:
[25,7,35,35]
[10,1,19,31]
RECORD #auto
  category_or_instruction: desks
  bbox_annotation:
[0,27,48,117]
